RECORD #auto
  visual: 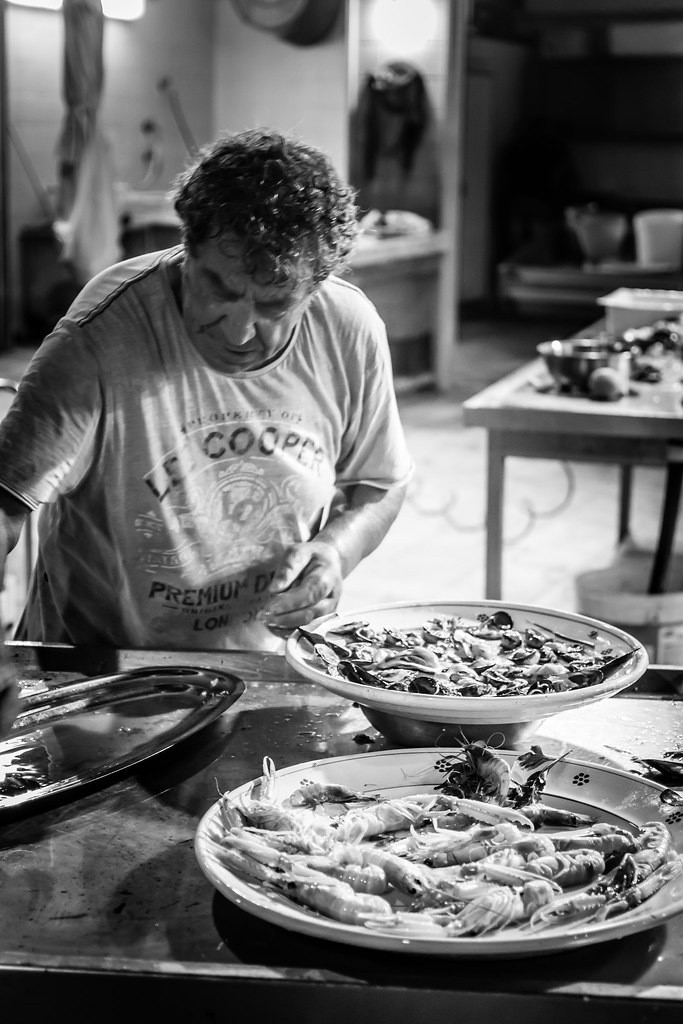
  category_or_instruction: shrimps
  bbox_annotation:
[204,728,683,935]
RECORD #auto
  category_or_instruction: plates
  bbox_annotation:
[194,749,683,953]
[286,601,648,725]
[1,665,245,810]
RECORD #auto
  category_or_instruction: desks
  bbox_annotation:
[0,684,683,1024]
[460,316,683,599]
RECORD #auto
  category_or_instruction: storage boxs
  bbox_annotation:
[598,287,683,333]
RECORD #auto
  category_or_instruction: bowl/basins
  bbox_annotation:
[536,339,639,393]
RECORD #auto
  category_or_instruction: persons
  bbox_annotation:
[0,128,415,655]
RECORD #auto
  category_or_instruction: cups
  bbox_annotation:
[635,211,683,265]
[576,214,627,268]
